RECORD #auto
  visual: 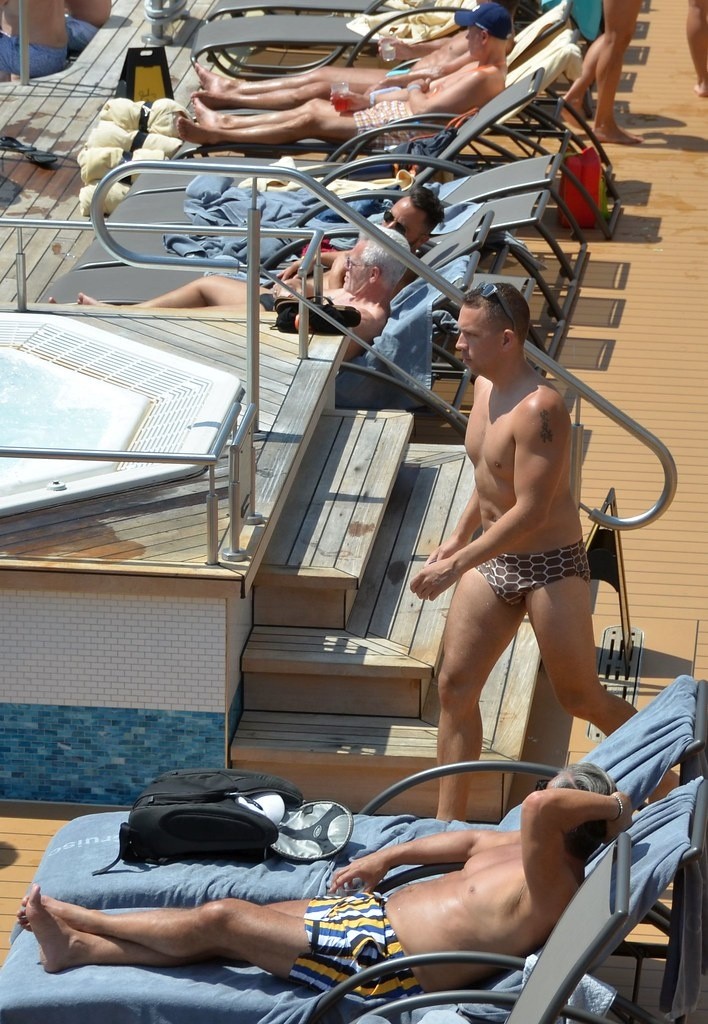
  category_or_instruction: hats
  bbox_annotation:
[454,3,513,39]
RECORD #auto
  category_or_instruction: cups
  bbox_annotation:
[331,82,349,113]
[380,34,396,62]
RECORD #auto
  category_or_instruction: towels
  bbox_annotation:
[78,97,193,215]
[10,672,699,935]
[542,0,603,41]
[505,0,568,64]
[163,155,484,264]
[522,954,619,1024]
[485,29,584,138]
[346,0,479,45]
[1,773,707,1024]
[336,253,472,407]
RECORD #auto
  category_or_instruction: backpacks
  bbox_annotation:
[118,768,304,863]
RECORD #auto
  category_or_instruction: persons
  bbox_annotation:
[63,0,111,54]
[47,188,446,363]
[687,0,708,97]
[0,0,68,81]
[178,0,517,145]
[16,763,632,1004]
[408,283,678,823]
[558,0,644,145]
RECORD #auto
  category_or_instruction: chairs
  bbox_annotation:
[44,0,626,385]
[0,673,708,1024]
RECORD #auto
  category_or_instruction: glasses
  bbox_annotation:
[383,210,406,236]
[346,257,372,269]
[476,280,517,331]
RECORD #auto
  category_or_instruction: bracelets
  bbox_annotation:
[369,94,375,109]
[407,84,421,92]
[607,793,623,822]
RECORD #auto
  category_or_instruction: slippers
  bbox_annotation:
[0,136,36,152]
[269,295,361,335]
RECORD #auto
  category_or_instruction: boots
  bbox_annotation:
[25,150,57,164]
